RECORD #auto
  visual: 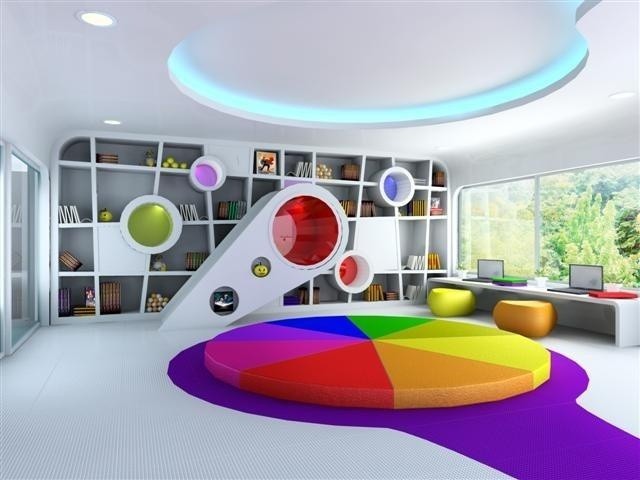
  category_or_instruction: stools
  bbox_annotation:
[492,298,558,338]
[427,288,477,319]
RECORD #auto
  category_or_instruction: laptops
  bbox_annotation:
[463,259,505,283]
[547,263,604,294]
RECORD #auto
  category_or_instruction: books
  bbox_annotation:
[217,200,247,220]
[297,287,309,304]
[313,286,320,304]
[100,281,122,314]
[428,253,441,270]
[186,252,209,270]
[341,164,359,180]
[340,200,355,216]
[60,287,72,314]
[364,284,385,301]
[360,200,377,217]
[407,199,425,216]
[255,150,277,176]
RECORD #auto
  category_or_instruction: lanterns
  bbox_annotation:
[99,207,113,222]
[254,262,268,277]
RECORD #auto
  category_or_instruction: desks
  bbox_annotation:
[428,277,640,347]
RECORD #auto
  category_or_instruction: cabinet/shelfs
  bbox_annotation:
[49,129,453,326]
